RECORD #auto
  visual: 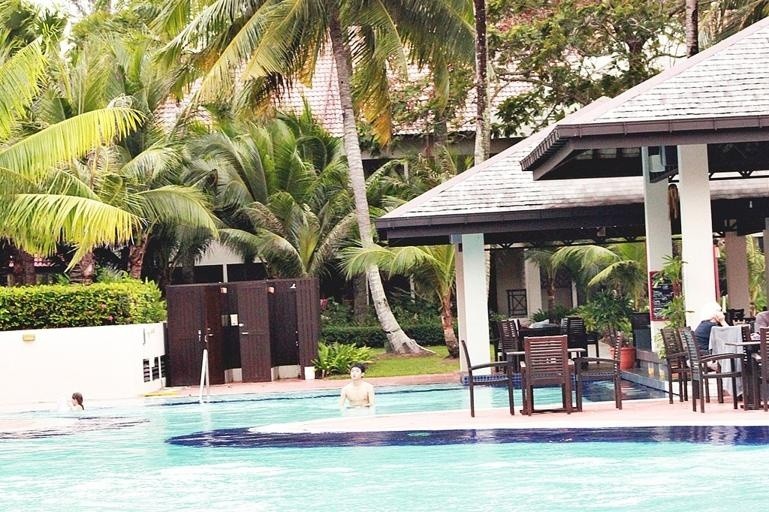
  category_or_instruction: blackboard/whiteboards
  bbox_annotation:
[649,271,680,321]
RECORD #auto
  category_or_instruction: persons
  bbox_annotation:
[67,393,85,412]
[686,303,729,370]
[339,363,375,409]
[747,302,757,315]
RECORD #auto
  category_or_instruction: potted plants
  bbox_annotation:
[575,289,637,372]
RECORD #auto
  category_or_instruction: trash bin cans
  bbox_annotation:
[632,313,651,352]
